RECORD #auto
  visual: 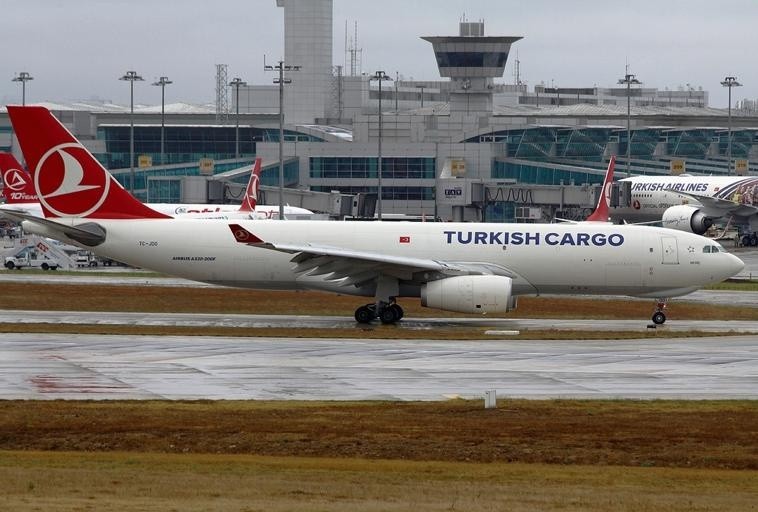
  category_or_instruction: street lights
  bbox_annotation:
[11,71,34,106]
[616,73,642,179]
[264,60,303,221]
[151,76,174,163]
[720,76,744,176]
[228,76,249,160]
[369,70,395,221]
[118,70,145,196]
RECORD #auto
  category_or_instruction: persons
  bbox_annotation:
[735,234,739,248]
